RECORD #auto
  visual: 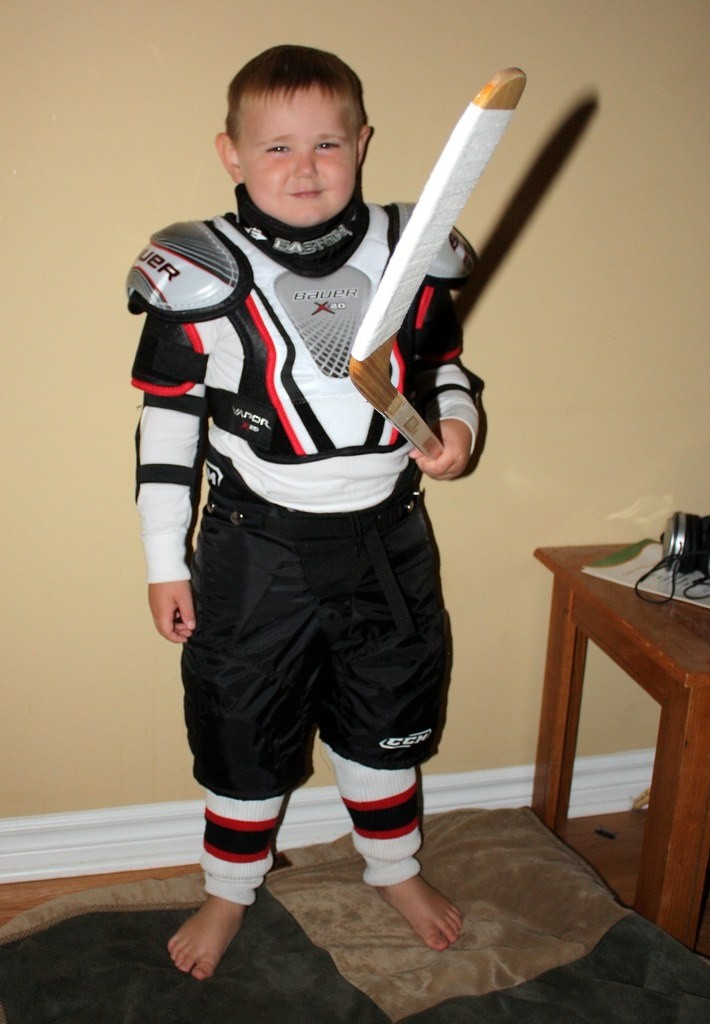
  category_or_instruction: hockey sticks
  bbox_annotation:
[347,66,529,461]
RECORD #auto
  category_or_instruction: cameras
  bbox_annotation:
[663,513,710,581]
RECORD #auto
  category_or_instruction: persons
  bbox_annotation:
[123,44,479,980]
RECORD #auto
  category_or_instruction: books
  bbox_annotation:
[581,538,710,609]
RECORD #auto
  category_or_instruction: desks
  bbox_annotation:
[534,540,710,953]
